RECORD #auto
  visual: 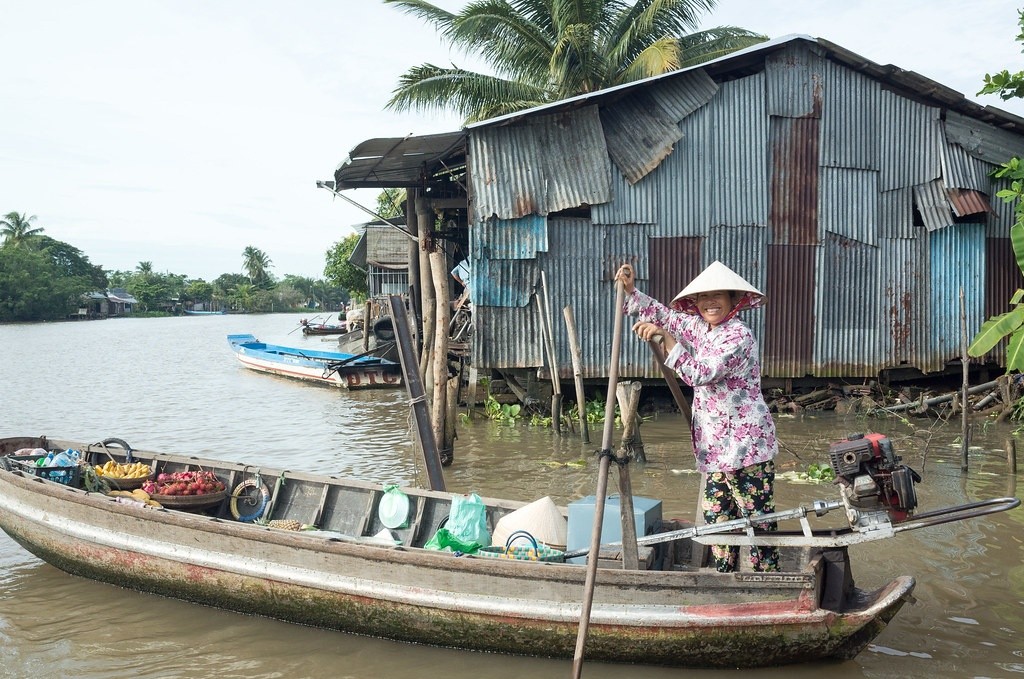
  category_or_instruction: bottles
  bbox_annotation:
[60,449,81,482]
[41,453,54,477]
[50,448,73,476]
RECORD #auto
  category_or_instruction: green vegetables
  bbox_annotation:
[460,381,654,427]
[534,459,588,468]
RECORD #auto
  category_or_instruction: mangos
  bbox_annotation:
[107,489,162,508]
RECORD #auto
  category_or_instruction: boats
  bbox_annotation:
[0,432,1021,671]
[184,310,223,316]
[227,333,403,390]
[300,318,348,335]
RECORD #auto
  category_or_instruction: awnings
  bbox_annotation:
[316,130,467,241]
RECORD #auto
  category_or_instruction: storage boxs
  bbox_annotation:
[567,494,663,569]
[6,454,80,490]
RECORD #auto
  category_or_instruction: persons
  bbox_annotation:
[613,261,778,573]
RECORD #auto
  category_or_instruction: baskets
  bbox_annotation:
[6,454,81,488]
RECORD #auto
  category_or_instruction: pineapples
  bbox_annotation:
[271,520,301,532]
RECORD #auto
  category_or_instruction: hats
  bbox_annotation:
[669,260,767,315]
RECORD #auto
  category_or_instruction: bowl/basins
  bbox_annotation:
[378,490,410,529]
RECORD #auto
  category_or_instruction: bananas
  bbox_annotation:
[95,460,152,479]
